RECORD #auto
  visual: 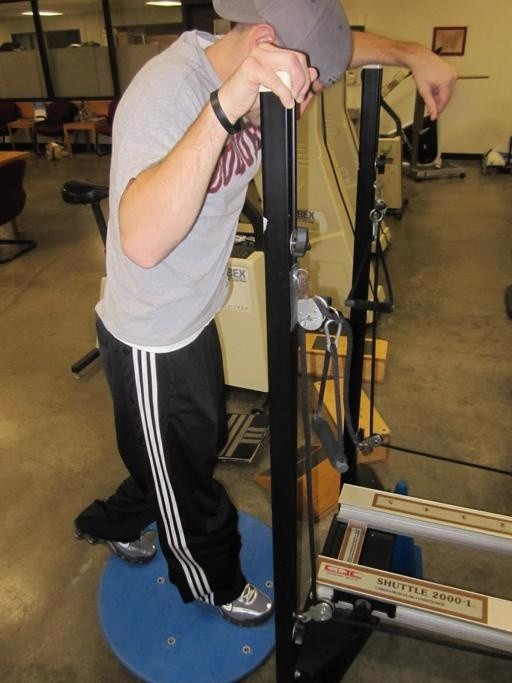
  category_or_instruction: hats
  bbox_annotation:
[212,0,352,88]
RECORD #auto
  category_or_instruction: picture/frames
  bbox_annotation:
[432,26,467,56]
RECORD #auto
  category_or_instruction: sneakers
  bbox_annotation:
[74,526,157,564]
[191,583,273,626]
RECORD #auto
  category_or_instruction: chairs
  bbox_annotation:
[0,164,36,263]
[33,101,78,155]
[0,101,22,150]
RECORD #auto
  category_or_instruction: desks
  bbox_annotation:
[0,150,32,164]
[7,118,45,154]
[63,115,107,156]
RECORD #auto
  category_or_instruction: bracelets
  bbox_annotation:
[209,88,241,136]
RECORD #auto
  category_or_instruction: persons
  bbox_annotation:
[72,0,458,628]
